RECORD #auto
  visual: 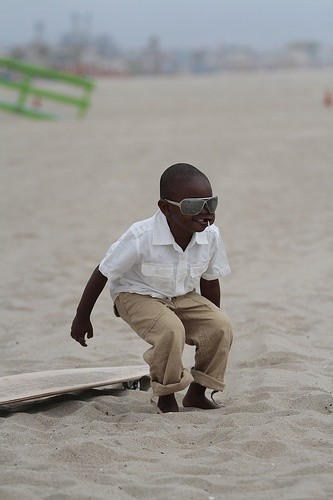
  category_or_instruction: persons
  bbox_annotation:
[68,163,235,415]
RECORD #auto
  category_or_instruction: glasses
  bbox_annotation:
[163,195,218,217]
